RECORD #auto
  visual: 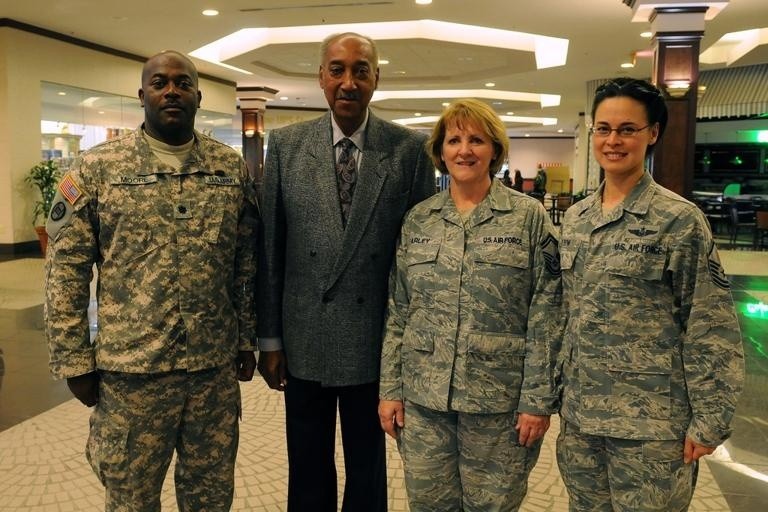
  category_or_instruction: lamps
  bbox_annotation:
[699,85,707,97]
[245,128,256,138]
[666,86,689,98]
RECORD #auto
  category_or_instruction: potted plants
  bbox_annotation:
[24,159,64,257]
[557,192,570,209]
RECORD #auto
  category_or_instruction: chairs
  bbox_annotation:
[695,197,768,250]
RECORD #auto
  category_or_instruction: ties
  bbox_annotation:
[335,138,358,228]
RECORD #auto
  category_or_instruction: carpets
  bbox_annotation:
[1,255,100,310]
[715,249,768,276]
[1,373,731,512]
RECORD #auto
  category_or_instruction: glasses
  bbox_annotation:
[592,124,650,137]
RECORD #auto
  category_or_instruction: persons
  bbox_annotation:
[531,163,546,191]
[510,169,523,193]
[43,46,261,511]
[500,169,512,189]
[256,31,435,512]
[373,96,567,510]
[554,75,745,511]
